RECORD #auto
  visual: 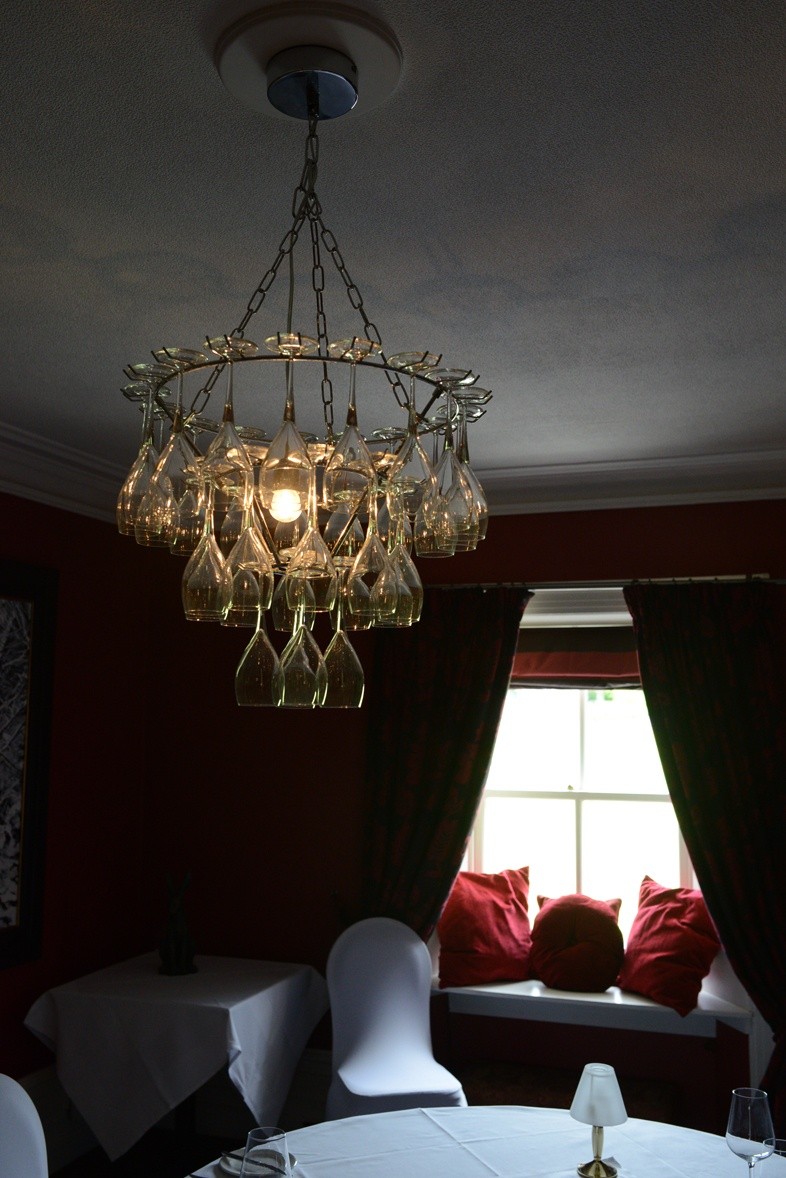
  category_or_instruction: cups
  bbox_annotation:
[239,1125,292,1178]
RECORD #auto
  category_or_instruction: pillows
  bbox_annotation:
[617,876,725,1017]
[429,860,537,992]
[527,892,627,996]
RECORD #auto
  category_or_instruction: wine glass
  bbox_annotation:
[104,330,494,712]
[726,1086,776,1178]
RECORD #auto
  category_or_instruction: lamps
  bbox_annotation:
[108,0,501,712]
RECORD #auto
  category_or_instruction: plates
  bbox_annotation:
[218,1147,298,1176]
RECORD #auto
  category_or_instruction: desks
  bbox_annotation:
[23,942,325,1165]
[179,1101,786,1178]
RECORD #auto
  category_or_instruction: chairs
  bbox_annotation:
[323,917,467,1117]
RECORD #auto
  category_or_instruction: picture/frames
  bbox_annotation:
[2,549,56,967]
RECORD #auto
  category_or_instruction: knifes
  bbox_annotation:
[220,1150,287,1176]
